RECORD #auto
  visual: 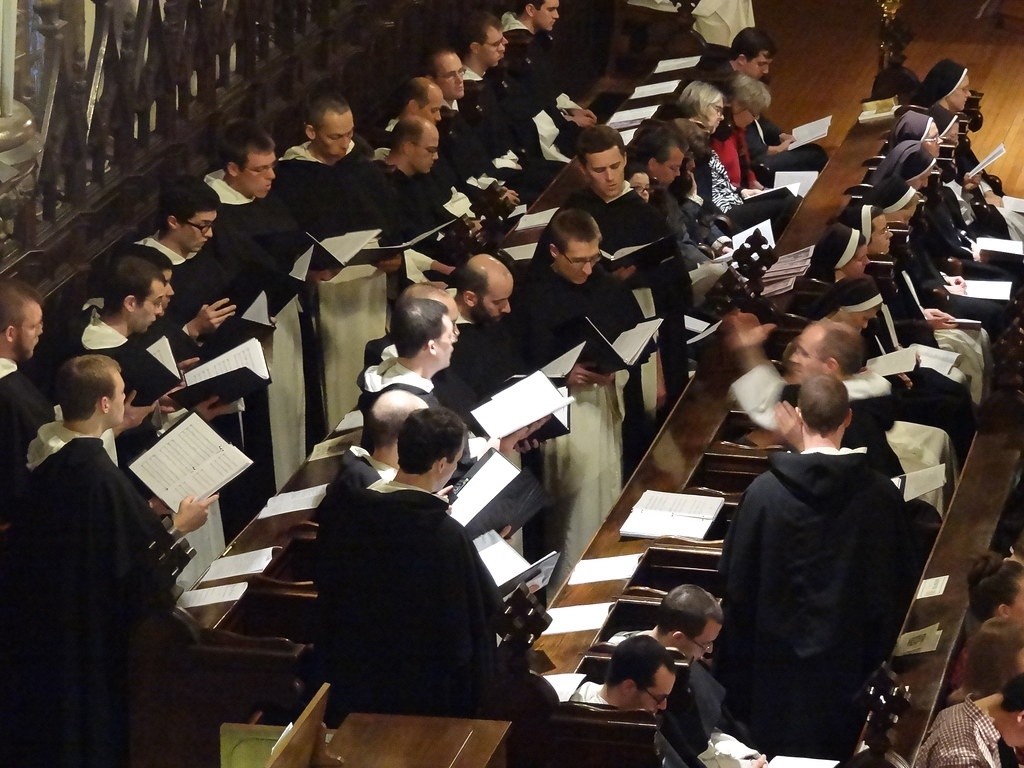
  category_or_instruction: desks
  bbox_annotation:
[326,714,512,768]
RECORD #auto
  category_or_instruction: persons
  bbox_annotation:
[149,0,1024,279]
[1,179,1024,768]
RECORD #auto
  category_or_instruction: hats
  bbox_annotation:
[838,272,884,312]
[811,223,860,283]
[894,109,933,146]
[840,205,873,246]
[927,104,958,137]
[910,59,968,108]
[853,174,917,213]
[869,140,933,187]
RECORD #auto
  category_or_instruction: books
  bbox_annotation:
[890,461,951,502]
[713,219,776,264]
[866,348,919,379]
[515,387,573,446]
[905,343,961,375]
[445,449,522,530]
[465,371,572,439]
[857,98,902,124]
[742,182,802,207]
[786,114,831,150]
[167,337,270,406]
[620,488,723,542]
[573,309,664,370]
[466,527,560,598]
[290,225,383,272]
[129,413,254,514]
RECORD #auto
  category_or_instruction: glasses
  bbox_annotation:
[644,688,669,704]
[691,639,713,651]
[750,112,760,117]
[562,248,602,265]
[243,161,278,175]
[630,186,654,193]
[924,134,939,142]
[715,106,724,116]
[485,37,503,47]
[879,225,890,235]
[434,67,467,80]
[185,221,216,233]
